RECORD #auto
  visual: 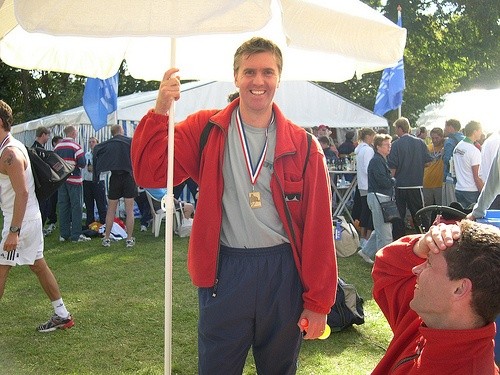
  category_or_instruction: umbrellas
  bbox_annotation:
[0,0,408,375]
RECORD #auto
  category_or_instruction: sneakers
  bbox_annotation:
[140,224,148,232]
[38,312,75,332]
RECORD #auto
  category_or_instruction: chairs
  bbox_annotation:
[145,190,195,237]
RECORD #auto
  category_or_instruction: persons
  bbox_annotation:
[131,36,338,375]
[52,126,91,243]
[92,123,137,247]
[0,100,74,332]
[29,125,199,236]
[305,120,500,250]
[371,219,500,375]
[358,133,396,265]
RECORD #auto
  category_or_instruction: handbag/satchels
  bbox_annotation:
[325,276,364,332]
[380,199,402,223]
[25,145,76,204]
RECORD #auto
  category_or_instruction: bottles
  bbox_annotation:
[87,159,92,172]
[300,318,331,340]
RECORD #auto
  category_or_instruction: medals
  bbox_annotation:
[249,192,261,209]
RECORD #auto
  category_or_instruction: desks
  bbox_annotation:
[328,171,359,224]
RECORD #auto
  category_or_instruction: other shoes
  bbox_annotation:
[42,224,57,235]
[126,237,135,247]
[59,235,66,242]
[358,248,374,264]
[359,238,368,248]
[73,234,91,242]
[101,239,111,246]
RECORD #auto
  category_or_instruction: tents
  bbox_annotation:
[8,80,396,220]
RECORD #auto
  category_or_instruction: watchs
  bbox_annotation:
[10,225,20,236]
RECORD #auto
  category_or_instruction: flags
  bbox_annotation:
[374,13,405,115]
[83,71,118,132]
